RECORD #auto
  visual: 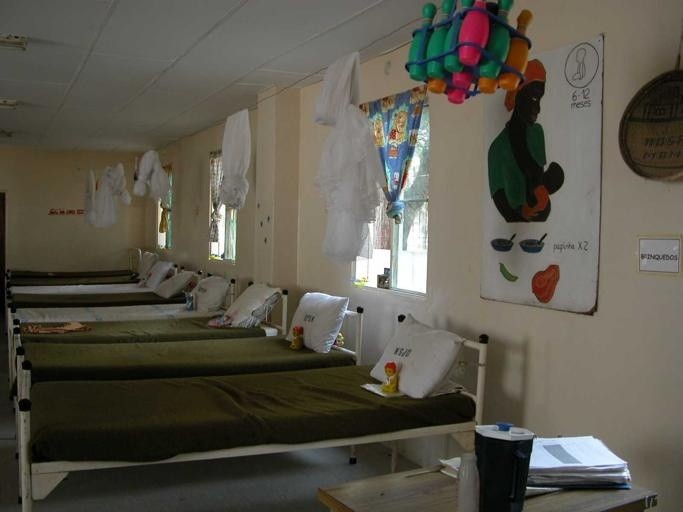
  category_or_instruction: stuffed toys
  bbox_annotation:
[289,326,306,350]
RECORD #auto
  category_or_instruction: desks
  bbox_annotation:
[316,454,657,512]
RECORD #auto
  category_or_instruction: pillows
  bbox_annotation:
[371,314,464,402]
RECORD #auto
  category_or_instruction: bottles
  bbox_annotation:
[455,444,481,512]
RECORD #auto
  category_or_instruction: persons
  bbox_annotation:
[381,362,400,393]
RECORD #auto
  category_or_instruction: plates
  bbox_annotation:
[491,238,514,252]
[518,238,545,253]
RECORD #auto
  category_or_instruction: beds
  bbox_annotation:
[2,248,365,391]
[15,313,490,512]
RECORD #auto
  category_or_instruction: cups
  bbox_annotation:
[377,272,388,289]
[474,423,537,512]
